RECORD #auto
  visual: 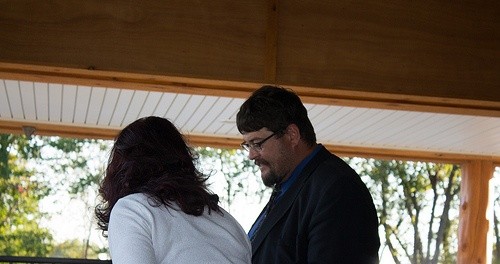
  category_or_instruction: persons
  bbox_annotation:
[236,86,381,264]
[94,116,252,264]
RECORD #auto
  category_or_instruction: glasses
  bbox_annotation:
[241,133,277,152]
